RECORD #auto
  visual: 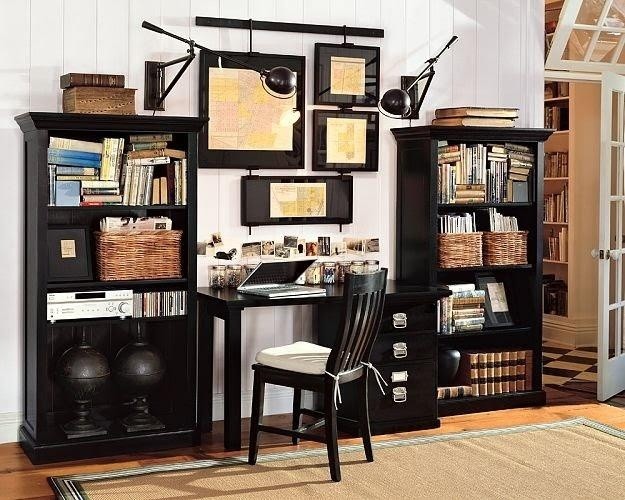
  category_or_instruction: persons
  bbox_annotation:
[305,242,317,256]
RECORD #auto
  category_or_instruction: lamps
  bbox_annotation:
[377,35,459,120]
[138,21,299,112]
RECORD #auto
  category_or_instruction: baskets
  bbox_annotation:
[484,231,530,266]
[94,229,184,282]
[438,231,484,268]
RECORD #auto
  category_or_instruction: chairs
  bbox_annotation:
[248,266,391,482]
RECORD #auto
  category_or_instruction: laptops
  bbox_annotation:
[236,257,327,296]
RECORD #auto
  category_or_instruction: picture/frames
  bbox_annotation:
[240,174,355,227]
[311,109,380,173]
[47,224,94,282]
[313,42,382,108]
[197,49,307,171]
[473,271,515,329]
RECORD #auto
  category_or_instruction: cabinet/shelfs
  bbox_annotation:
[544,3,602,351]
[12,110,211,466]
[389,125,559,418]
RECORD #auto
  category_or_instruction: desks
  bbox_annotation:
[197,275,454,452]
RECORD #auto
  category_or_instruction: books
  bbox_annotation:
[430,107,519,128]
[132,289,185,319]
[58,71,124,88]
[43,132,188,207]
[542,81,570,319]
[437,139,536,398]
[61,87,139,114]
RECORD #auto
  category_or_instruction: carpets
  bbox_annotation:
[44,413,624,499]
[542,347,616,387]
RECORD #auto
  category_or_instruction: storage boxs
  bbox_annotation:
[62,86,137,116]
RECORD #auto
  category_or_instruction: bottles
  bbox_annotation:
[306,261,322,285]
[365,260,380,275]
[242,263,258,284]
[225,264,243,288]
[335,261,351,283]
[320,262,337,284]
[352,261,365,276]
[209,263,227,291]
[295,271,308,286]
